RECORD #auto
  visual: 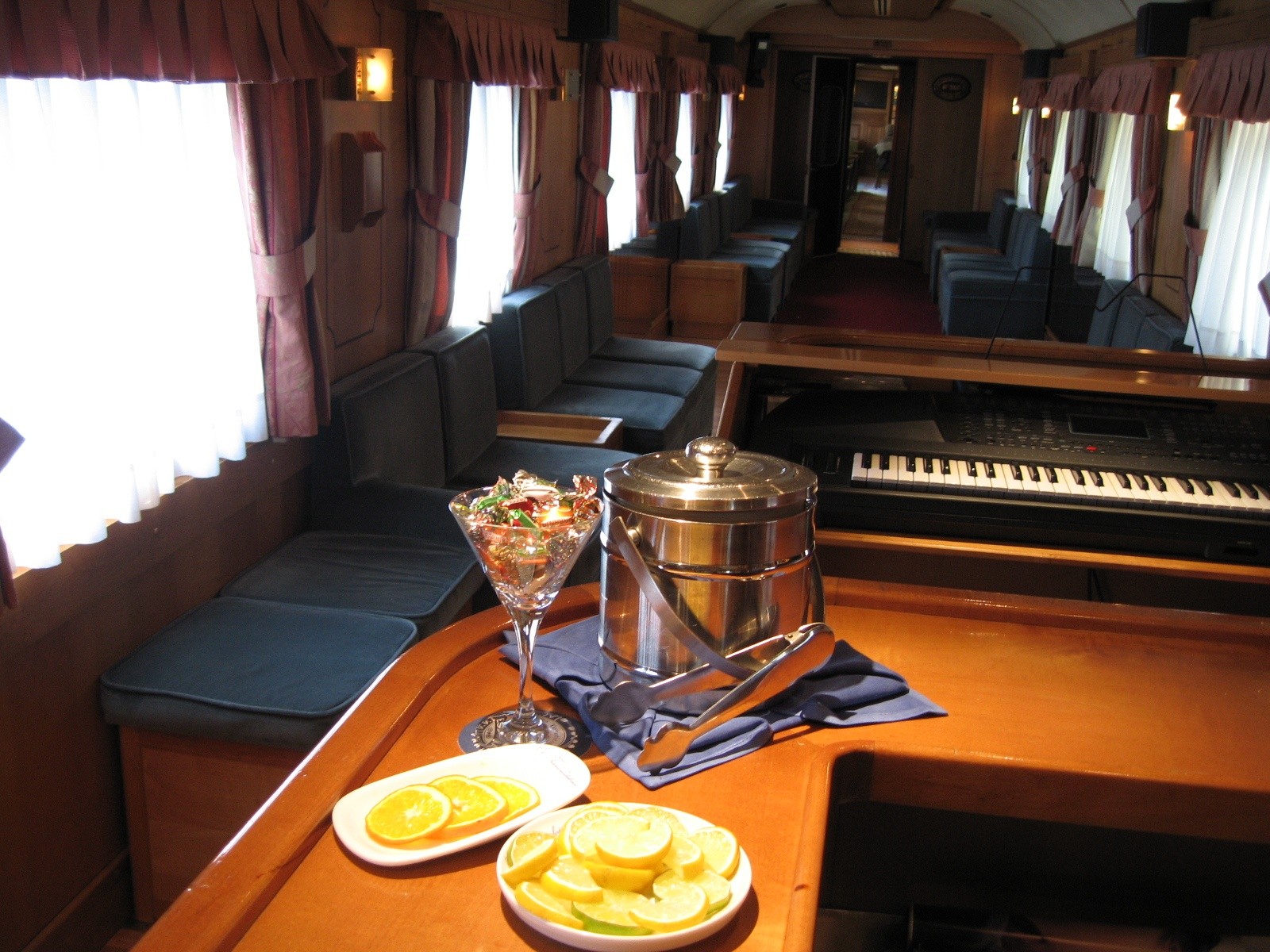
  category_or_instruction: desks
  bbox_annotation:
[669,258,748,340]
[607,255,672,340]
[127,572,1270,952]
[494,407,624,449]
[730,232,772,241]
[937,246,999,295]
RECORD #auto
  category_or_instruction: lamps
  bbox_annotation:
[560,68,581,101]
[355,46,394,102]
[1167,94,1195,131]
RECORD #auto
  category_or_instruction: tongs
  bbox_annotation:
[591,622,834,776]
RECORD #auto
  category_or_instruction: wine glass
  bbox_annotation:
[448,485,606,752]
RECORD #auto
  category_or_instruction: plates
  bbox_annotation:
[496,800,753,951]
[330,743,593,868]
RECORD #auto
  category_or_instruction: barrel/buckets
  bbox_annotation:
[599,434,827,682]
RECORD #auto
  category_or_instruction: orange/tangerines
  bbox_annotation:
[501,801,739,937]
[365,773,540,846]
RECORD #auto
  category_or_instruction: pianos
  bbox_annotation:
[795,436,1270,568]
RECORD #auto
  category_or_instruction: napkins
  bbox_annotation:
[498,614,948,791]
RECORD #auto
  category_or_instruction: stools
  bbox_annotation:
[100,527,483,927]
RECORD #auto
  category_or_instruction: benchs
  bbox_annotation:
[314,178,806,618]
[925,188,1185,351]
